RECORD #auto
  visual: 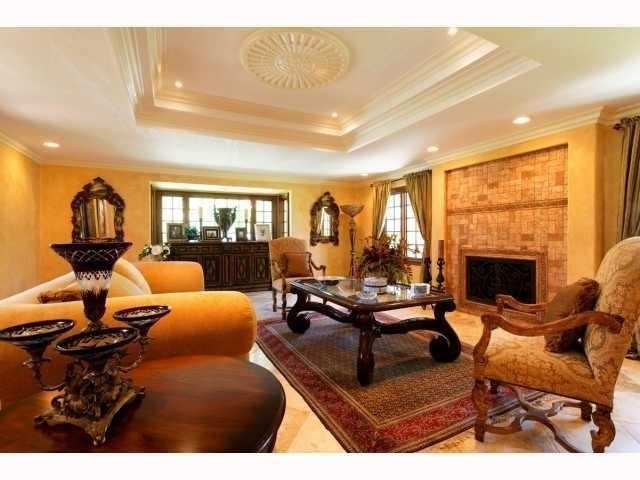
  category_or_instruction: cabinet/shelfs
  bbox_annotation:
[162,241,272,292]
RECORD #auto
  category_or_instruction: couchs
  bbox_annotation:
[267,237,326,319]
[0,261,265,406]
[470,235,640,448]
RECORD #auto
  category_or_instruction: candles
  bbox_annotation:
[424,240,431,257]
[438,240,445,258]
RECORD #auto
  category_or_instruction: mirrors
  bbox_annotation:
[309,190,340,246]
[70,177,125,243]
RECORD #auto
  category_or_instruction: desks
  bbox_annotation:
[1,356,287,453]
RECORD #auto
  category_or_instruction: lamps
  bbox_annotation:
[338,204,361,276]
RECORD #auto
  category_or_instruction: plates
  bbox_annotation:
[56,327,138,372]
[0,320,75,360]
[113,305,172,338]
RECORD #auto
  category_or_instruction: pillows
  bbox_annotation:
[113,257,152,295]
[36,271,146,304]
[283,252,314,278]
[541,278,600,353]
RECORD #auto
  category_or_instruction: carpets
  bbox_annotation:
[254,308,550,451]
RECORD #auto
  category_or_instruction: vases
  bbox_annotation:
[364,272,389,294]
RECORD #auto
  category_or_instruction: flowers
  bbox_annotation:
[351,234,419,285]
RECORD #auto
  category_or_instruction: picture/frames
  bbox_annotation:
[203,226,221,240]
[253,224,270,241]
[167,223,184,242]
[236,227,247,241]
